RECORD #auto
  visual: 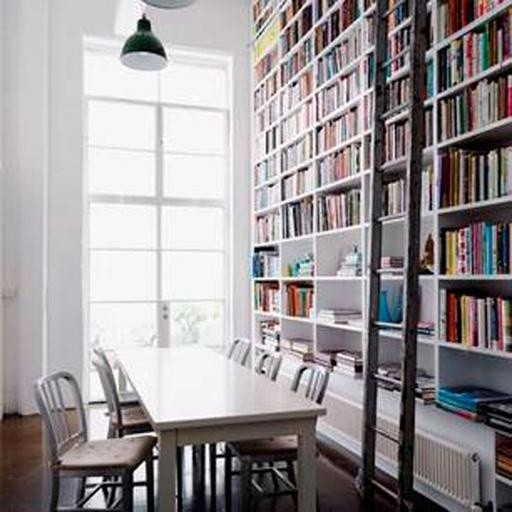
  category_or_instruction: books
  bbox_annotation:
[262,319,279,348]
[283,252,313,274]
[434,386,509,430]
[285,282,312,317]
[386,58,433,117]
[496,444,512,479]
[257,167,278,210]
[313,138,371,189]
[254,214,279,242]
[253,93,280,136]
[284,336,311,362]
[441,220,509,276]
[283,102,308,140]
[281,37,309,84]
[315,0,338,24]
[283,129,312,176]
[320,307,366,325]
[433,0,506,43]
[439,287,512,353]
[282,8,311,58]
[255,45,278,91]
[313,17,370,88]
[252,0,281,37]
[335,251,364,276]
[312,58,374,124]
[386,1,409,34]
[253,250,276,275]
[440,145,512,208]
[387,107,434,168]
[313,0,375,57]
[254,13,277,66]
[283,197,317,241]
[314,107,374,178]
[280,0,311,31]
[255,281,277,310]
[378,256,405,274]
[380,163,438,218]
[383,26,433,82]
[437,9,512,98]
[373,360,436,403]
[285,164,312,202]
[279,74,311,126]
[319,184,362,232]
[257,66,278,114]
[256,121,280,162]
[435,75,512,146]
[254,150,279,186]
[313,350,362,375]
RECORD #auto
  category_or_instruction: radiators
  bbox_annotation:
[375,416,479,512]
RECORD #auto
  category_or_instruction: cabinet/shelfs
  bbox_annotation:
[248,0,512,512]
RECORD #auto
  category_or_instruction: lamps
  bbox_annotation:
[120,0,170,70]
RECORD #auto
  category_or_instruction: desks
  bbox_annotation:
[110,346,326,511]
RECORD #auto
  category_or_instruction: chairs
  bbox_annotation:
[92,358,184,512]
[209,350,282,511]
[36,371,159,512]
[94,348,144,406]
[225,338,250,364]
[226,361,328,511]
